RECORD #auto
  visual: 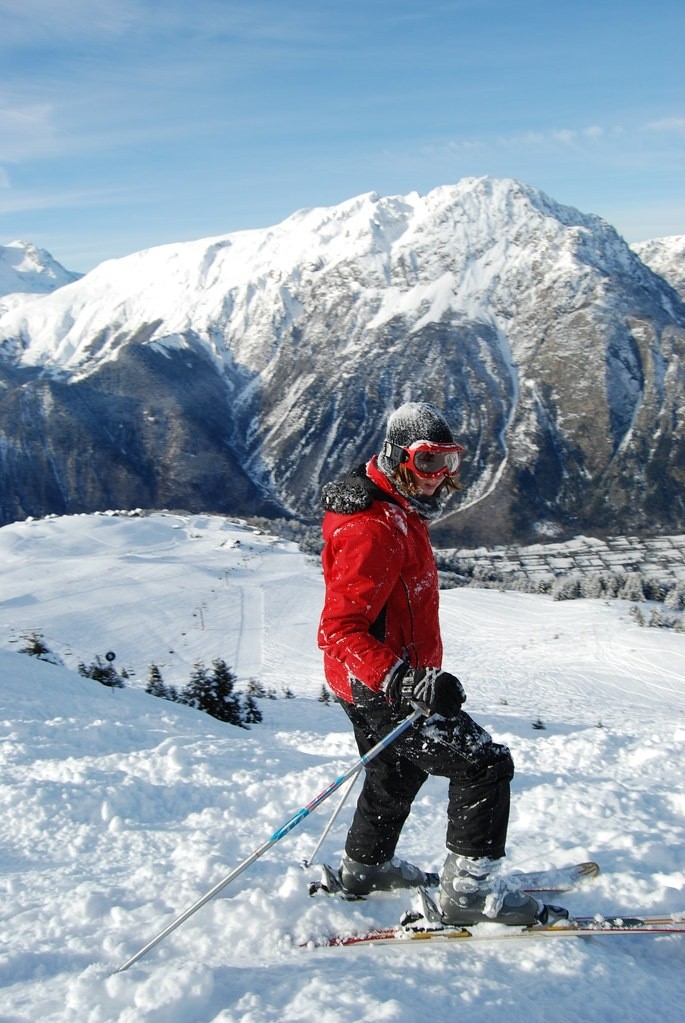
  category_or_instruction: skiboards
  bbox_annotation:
[294,855,685,954]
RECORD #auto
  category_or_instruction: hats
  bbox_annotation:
[380,402,454,477]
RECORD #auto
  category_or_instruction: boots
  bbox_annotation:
[437,852,540,926]
[336,854,429,896]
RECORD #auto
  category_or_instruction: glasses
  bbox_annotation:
[400,441,462,479]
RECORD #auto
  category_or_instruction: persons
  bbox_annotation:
[317,402,570,927]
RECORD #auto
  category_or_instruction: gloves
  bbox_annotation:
[386,662,467,718]
